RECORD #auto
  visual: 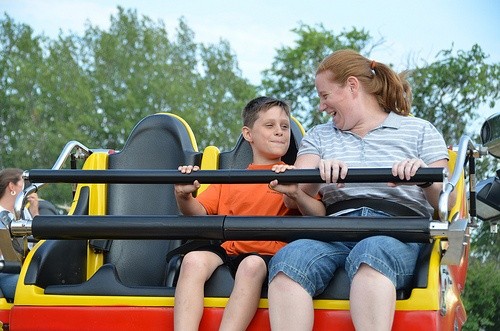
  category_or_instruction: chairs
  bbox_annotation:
[13,112,464,310]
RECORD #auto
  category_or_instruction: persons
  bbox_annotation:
[0,167,41,266]
[268,50,449,331]
[173,95,326,331]
